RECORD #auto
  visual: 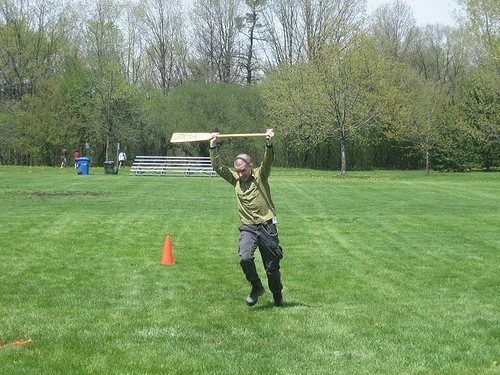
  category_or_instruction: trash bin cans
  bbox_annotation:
[75,157,91,175]
[104,161,115,174]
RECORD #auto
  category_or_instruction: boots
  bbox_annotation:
[246,279,265,306]
[272,292,286,307]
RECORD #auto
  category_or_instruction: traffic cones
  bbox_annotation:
[160,235,174,266]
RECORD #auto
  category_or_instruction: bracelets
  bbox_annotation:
[210,146,216,149]
[266,143,272,148]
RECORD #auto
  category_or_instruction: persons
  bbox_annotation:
[60,146,70,168]
[73,150,80,170]
[118,149,127,169]
[209,129,283,307]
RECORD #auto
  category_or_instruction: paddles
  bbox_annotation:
[170,132,274,143]
